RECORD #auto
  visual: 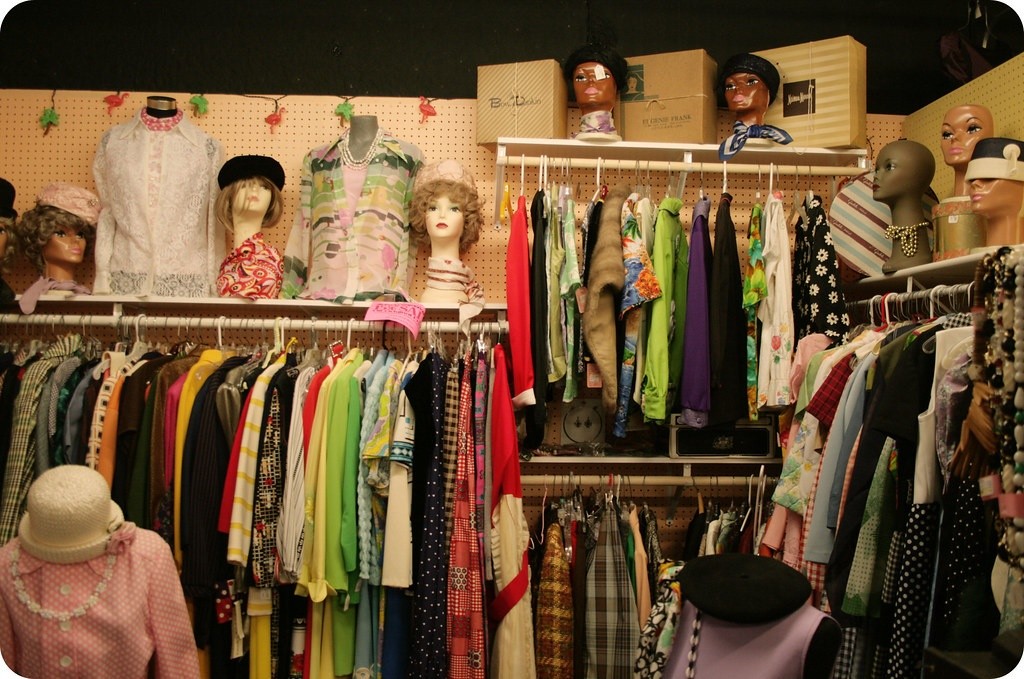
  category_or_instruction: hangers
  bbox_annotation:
[0,312,502,387]
[496,157,815,231]
[858,280,974,336]
[528,471,779,550]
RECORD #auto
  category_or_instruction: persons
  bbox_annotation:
[562,42,630,141]
[214,155,285,299]
[941,104,994,197]
[407,161,487,308]
[633,558,843,679]
[279,114,420,303]
[0,464,201,679]
[0,154,103,299]
[92,96,229,299]
[872,140,936,274]
[715,51,780,138]
[963,136,1024,246]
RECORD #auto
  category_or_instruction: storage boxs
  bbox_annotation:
[476,58,571,152]
[750,35,868,149]
[660,413,776,459]
[613,48,720,144]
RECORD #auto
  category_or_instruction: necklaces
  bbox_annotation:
[140,107,183,131]
[11,540,116,620]
[340,127,384,171]
[884,221,929,257]
[972,246,1024,579]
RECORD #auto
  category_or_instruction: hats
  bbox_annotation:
[964,136,1024,183]
[37,180,103,230]
[218,155,285,192]
[677,551,831,626]
[0,177,19,219]
[17,464,137,563]
[413,159,477,195]
[564,40,640,89]
[719,53,781,106]
